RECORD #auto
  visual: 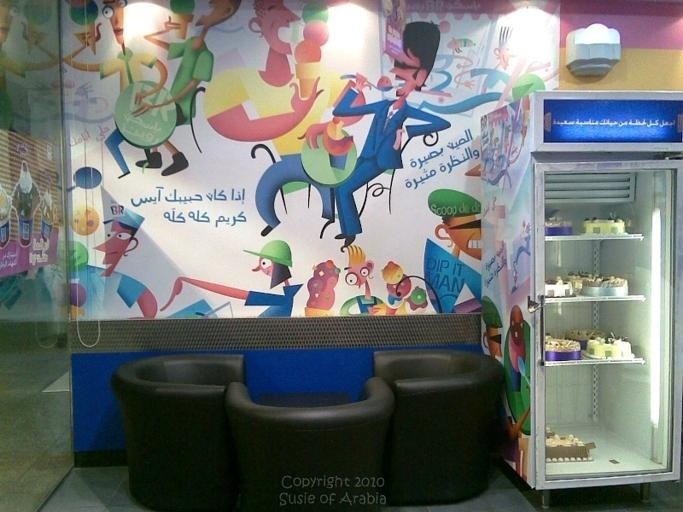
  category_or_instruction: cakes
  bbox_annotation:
[545,276,576,298]
[585,338,635,360]
[543,336,581,361]
[582,275,629,298]
[546,427,593,463]
[544,214,572,236]
[566,271,598,291]
[567,329,609,349]
[581,216,628,236]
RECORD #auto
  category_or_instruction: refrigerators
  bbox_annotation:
[480,89,683,507]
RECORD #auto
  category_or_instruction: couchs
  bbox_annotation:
[374,350,507,506]
[227,374,396,512]
[110,353,245,512]
